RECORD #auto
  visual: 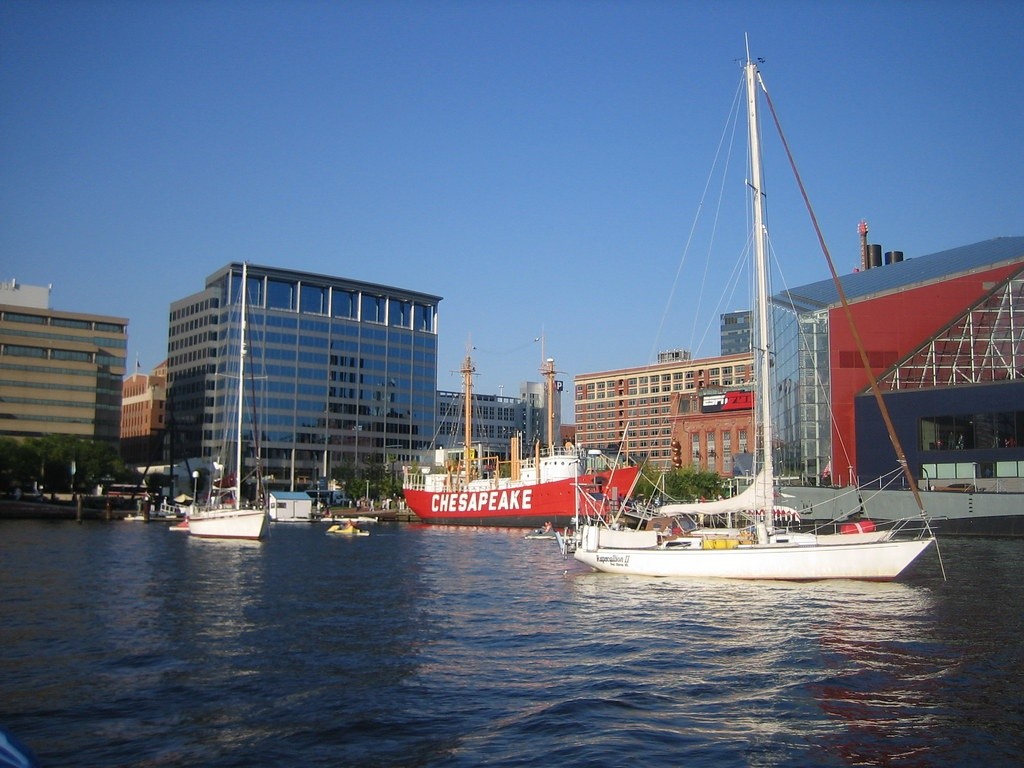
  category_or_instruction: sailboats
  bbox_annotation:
[185,262,270,542]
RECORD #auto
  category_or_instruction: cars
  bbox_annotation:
[934,483,977,492]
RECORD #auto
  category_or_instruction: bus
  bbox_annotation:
[97,484,160,502]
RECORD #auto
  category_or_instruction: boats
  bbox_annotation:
[124,513,148,523]
[573,31,947,581]
[326,525,370,537]
[555,455,617,556]
[524,521,567,540]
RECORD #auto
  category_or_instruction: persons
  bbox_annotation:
[354,492,402,513]
[541,521,553,535]
[618,493,725,507]
[947,431,966,449]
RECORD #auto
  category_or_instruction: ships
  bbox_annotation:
[402,329,640,529]
[284,340,420,460]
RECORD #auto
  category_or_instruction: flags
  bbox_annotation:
[822,461,830,480]
